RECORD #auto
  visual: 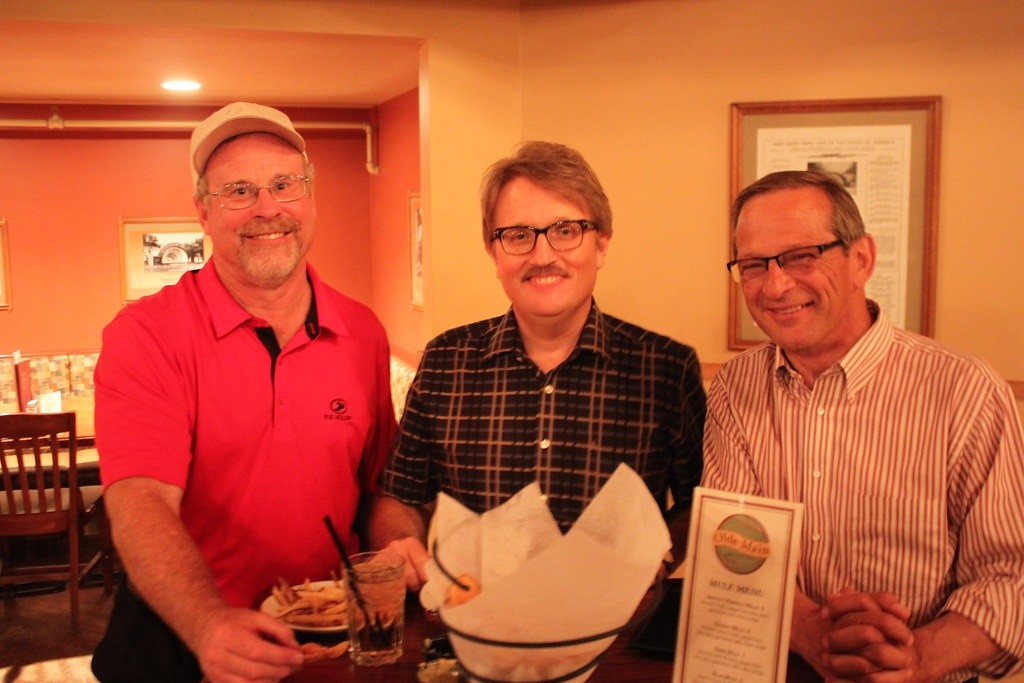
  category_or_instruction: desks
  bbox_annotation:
[201,606,821,683]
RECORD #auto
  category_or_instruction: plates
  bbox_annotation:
[260,579,347,633]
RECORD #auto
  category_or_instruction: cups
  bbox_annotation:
[343,549,407,665]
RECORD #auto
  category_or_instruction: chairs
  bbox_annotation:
[0,412,109,635]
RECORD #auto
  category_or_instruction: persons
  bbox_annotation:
[91,103,397,683]
[702,171,1024,683]
[368,143,707,592]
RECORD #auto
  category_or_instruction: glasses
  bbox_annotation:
[206,175,310,210]
[726,239,845,283]
[491,219,598,255]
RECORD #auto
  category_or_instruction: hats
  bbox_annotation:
[190,101,309,186]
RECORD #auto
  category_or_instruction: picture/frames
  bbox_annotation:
[0,218,13,310]
[409,195,423,310]
[118,216,213,306]
[725,95,942,353]
